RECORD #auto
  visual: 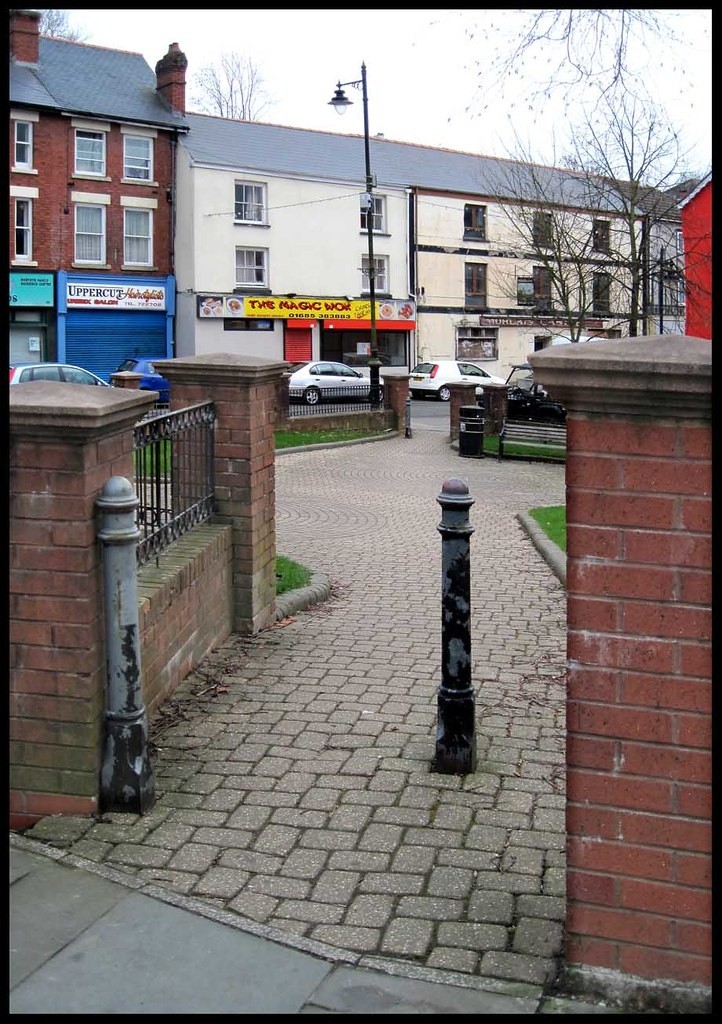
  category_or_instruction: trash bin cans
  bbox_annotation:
[458,405,486,458]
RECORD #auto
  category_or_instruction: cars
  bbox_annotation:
[502,364,566,423]
[9,361,117,388]
[407,359,509,402]
[281,359,385,405]
[107,356,173,406]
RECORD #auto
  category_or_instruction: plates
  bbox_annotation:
[201,298,223,317]
[227,299,243,313]
[380,303,395,319]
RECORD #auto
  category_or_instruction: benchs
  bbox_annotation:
[497,416,567,463]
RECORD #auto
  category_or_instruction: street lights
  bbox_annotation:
[325,59,385,408]
